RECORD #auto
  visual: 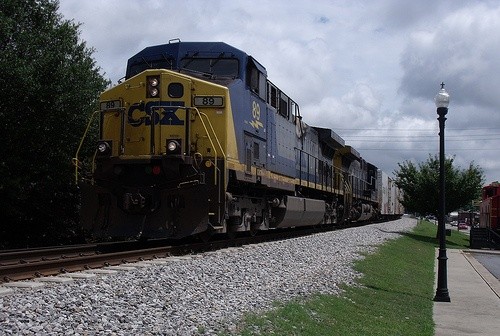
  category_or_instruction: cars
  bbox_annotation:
[449,220,458,226]
[425,213,434,219]
[458,223,468,230]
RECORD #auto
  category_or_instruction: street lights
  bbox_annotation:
[433,80,455,302]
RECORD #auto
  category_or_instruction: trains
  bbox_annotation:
[70,37,406,250]
